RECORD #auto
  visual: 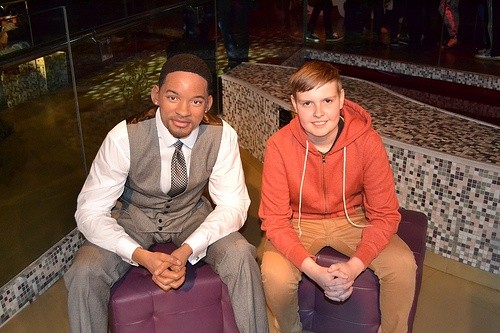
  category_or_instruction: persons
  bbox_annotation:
[185,1,495,58]
[63,52,268,333]
[258,60,417,333]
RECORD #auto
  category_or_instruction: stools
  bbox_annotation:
[108,242,239,333]
[298,209,428,333]
